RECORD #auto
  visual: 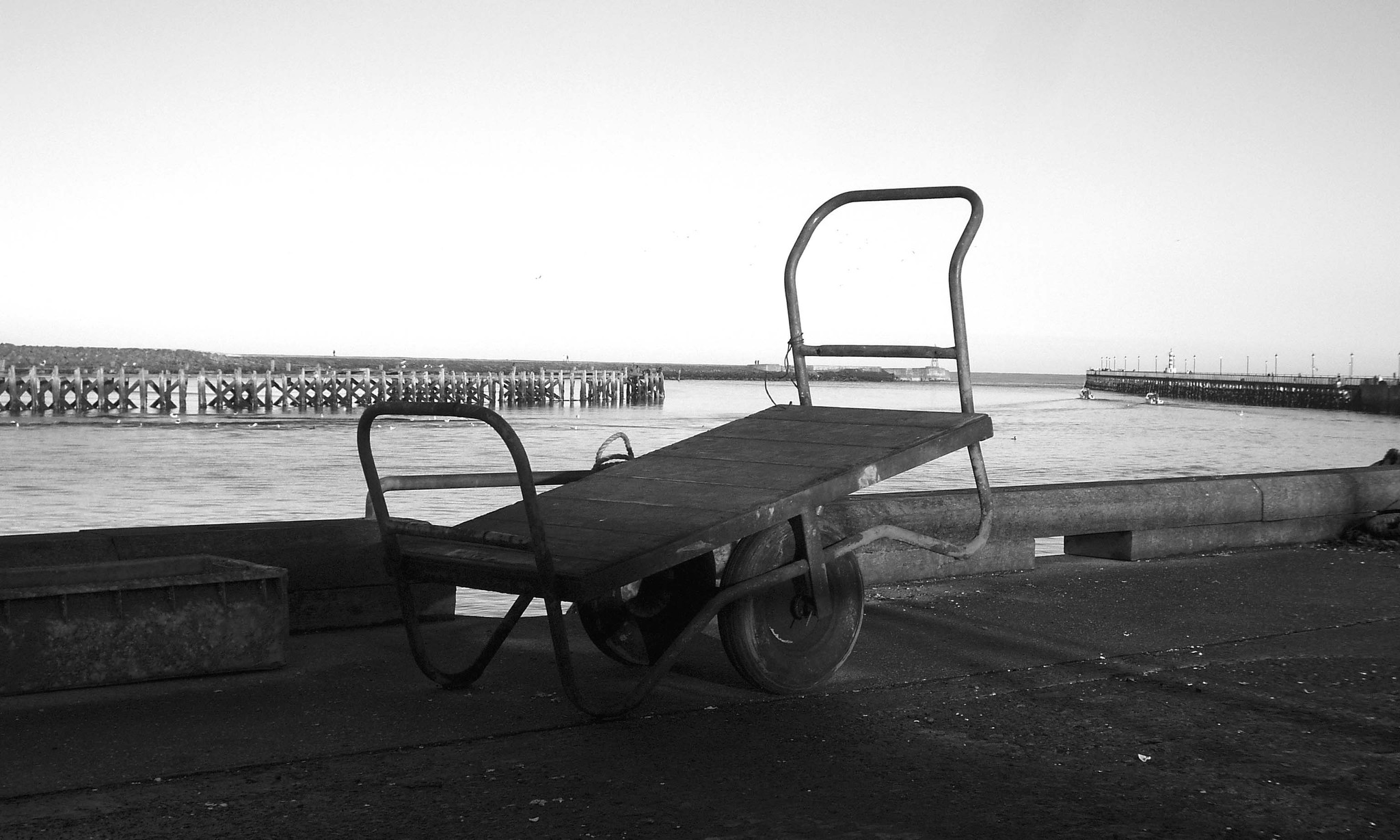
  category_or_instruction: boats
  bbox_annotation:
[1079,387,1095,400]
[1145,391,1164,405]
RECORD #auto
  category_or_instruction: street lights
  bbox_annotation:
[1125,356,1127,372]
[1350,353,1354,378]
[1312,354,1314,378]
[1138,356,1140,372]
[1398,353,1400,378]
[1185,359,1187,374]
[1265,361,1268,376]
[1274,354,1277,377]
[1114,357,1116,372]
[1101,357,1103,370]
[1106,357,1107,369]
[1155,355,1158,373]
[1193,355,1196,374]
[1108,357,1110,369]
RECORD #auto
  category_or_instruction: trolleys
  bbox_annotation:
[355,185,995,722]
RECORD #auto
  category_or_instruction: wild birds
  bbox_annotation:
[175,418,181,424]
[568,426,578,430]
[275,424,280,428]
[169,411,178,417]
[550,424,557,427]
[10,421,19,427]
[470,422,475,427]
[320,413,324,418]
[445,418,451,423]
[1239,410,1244,416]
[215,423,219,428]
[138,423,142,428]
[247,423,258,428]
[1010,436,1016,440]
[233,412,238,415]
[574,414,580,418]
[434,415,436,420]
[116,419,121,426]
[390,426,396,429]
[410,417,414,421]
[309,426,316,430]
